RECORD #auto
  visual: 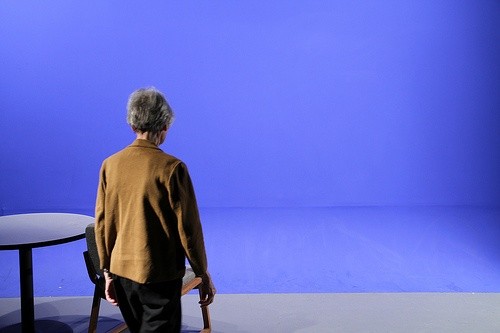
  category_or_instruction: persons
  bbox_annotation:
[94,89,216,333]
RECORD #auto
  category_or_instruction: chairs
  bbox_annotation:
[83,224,212,333]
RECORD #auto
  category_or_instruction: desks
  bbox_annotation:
[0,213,95,333]
